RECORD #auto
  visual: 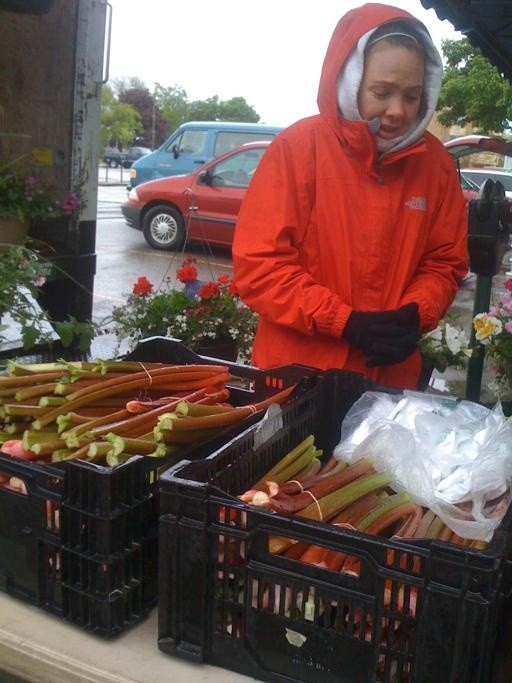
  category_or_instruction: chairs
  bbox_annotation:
[476,177,506,203]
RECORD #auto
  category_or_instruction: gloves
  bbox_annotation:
[343,302,422,368]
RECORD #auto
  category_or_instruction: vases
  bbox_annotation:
[190,340,240,366]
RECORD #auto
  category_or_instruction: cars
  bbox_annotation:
[444,134,512,206]
[103,120,287,250]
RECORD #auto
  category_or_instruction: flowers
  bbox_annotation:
[473,270,512,398]
[417,310,470,373]
[2,135,98,351]
[93,246,260,356]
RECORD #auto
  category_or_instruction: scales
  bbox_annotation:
[424,178,512,416]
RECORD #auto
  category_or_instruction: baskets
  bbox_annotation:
[0,332,510,682]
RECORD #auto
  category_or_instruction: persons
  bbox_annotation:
[231,3,469,390]
